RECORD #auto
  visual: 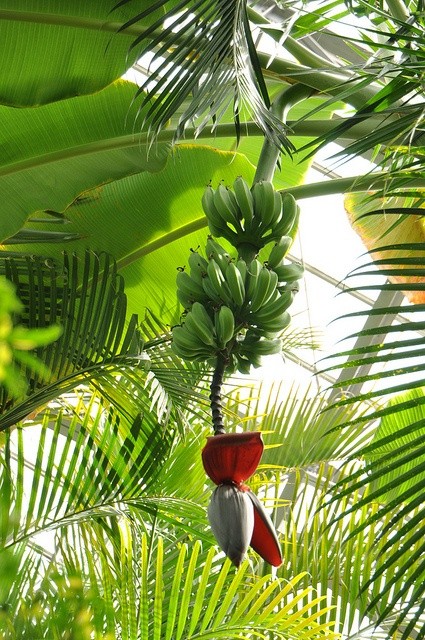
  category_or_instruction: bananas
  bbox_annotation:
[170,302,283,374]
[172,236,304,335]
[201,179,300,245]
